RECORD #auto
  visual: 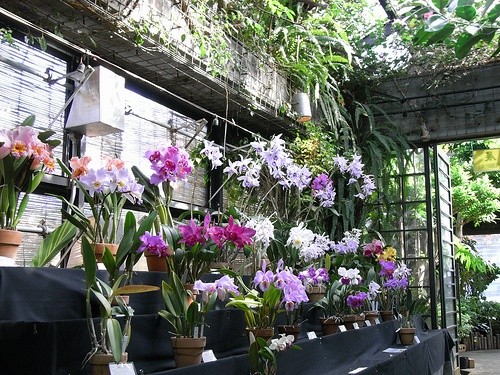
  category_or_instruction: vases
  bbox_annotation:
[341,317,355,330]
[319,319,339,336]
[346,315,364,328]
[144,246,173,272]
[363,312,376,326]
[380,311,392,322]
[91,244,119,263]
[0,229,22,261]
[307,287,325,303]
[170,336,206,367]
[278,325,300,344]
[396,328,416,344]
[89,352,127,375]
[0,114,430,375]
[245,327,274,346]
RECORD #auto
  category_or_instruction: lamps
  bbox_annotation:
[286,93,312,122]
